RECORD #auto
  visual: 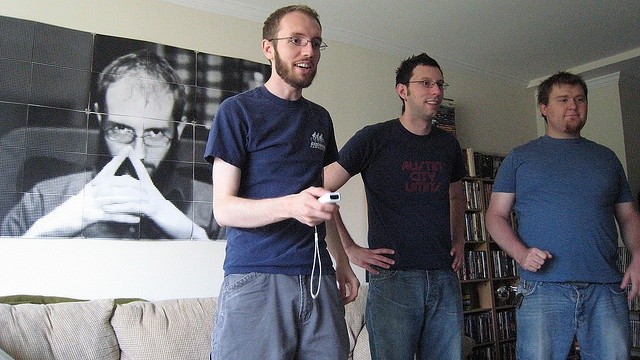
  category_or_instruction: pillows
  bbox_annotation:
[343,283,370,359]
[353,323,476,359]
[109,296,218,359]
[0,296,120,359]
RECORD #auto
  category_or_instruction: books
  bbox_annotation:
[492,251,516,277]
[463,313,494,343]
[630,321,640,348]
[464,250,488,280]
[496,286,518,305]
[463,286,479,310]
[501,344,515,359]
[616,248,631,273]
[626,285,639,311]
[498,311,517,339]
[465,212,483,242]
[471,348,495,359]
[464,179,482,210]
[435,100,455,133]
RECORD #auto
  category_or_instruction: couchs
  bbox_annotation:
[1,283,476,358]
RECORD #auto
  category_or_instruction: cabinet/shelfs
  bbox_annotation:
[613,246,639,359]
[447,147,579,360]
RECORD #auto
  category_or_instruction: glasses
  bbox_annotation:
[409,80,449,91]
[105,121,175,148]
[268,35,328,52]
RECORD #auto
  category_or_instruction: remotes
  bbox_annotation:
[315,192,341,203]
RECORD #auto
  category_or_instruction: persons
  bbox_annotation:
[0,53,227,240]
[485,70,636,358]
[204,6,360,358]
[323,52,467,360]
